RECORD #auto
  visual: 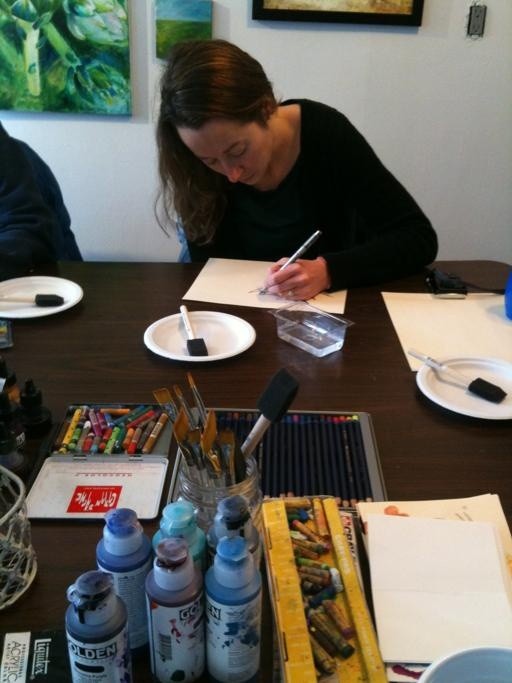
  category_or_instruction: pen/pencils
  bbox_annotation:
[258,231,322,294]
[213,411,373,508]
[286,498,353,678]
[53,405,169,457]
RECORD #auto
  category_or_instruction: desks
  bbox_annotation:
[0,260,511,683]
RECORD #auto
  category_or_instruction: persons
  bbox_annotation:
[1,112,83,265]
[154,36,440,305]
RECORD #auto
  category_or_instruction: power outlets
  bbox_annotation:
[467,6,486,35]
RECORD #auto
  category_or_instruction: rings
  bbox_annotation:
[287,290,297,301]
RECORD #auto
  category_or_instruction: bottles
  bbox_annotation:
[65,571,132,682]
[152,502,207,574]
[146,537,204,682]
[206,496,262,573]
[0,359,53,490]
[174,452,263,528]
[96,507,150,650]
[206,535,263,682]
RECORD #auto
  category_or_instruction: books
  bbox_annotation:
[351,490,512,682]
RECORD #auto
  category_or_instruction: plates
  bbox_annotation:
[1,275,84,319]
[143,310,256,362]
[415,355,511,420]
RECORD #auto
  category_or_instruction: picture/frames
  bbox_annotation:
[252,0,425,26]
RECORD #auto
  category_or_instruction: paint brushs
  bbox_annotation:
[153,373,236,489]
